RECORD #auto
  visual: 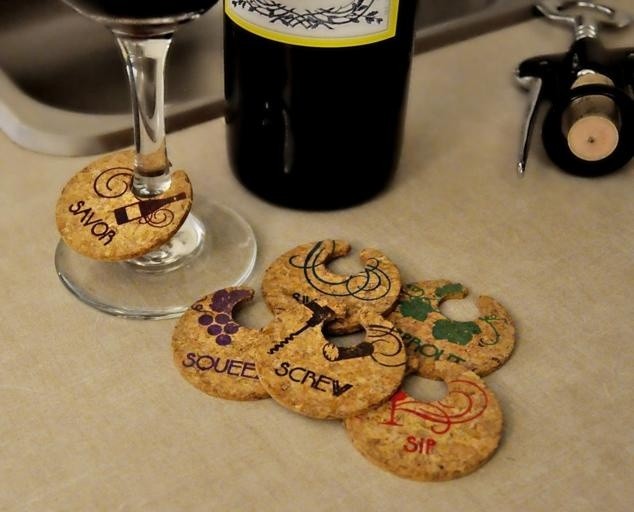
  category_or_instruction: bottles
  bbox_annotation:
[215,0,416,213]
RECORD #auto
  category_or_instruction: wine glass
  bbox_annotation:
[52,1,256,319]
